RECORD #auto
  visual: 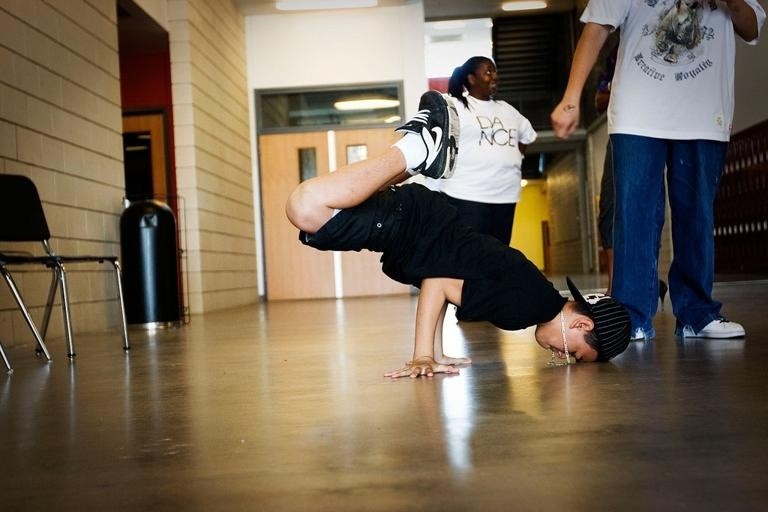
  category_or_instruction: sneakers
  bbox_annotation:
[394,90,461,179]
[682,316,745,338]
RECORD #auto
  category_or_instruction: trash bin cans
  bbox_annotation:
[120,198,181,331]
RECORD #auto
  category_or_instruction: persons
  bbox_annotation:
[438,57,537,323]
[595,42,620,297]
[550,0,767,339]
[285,91,632,378]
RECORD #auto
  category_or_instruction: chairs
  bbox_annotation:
[0,261,53,375]
[0,173,130,359]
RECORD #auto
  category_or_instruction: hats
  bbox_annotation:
[567,277,631,363]
